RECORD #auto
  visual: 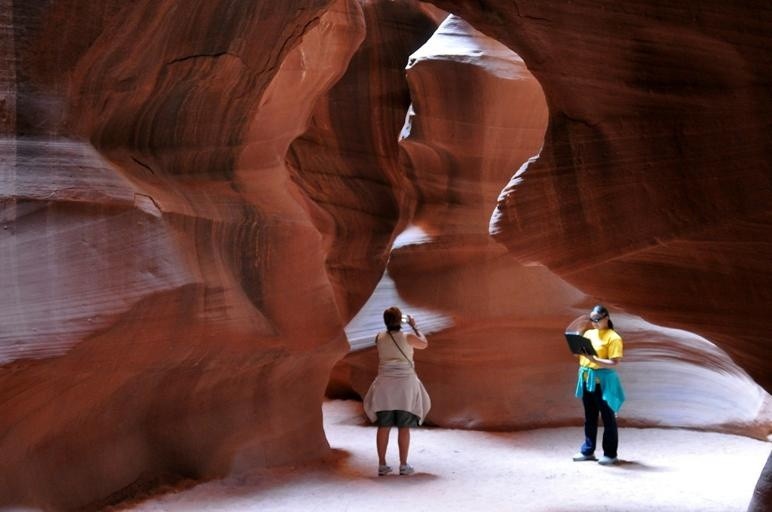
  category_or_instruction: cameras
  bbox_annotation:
[400,314,410,323]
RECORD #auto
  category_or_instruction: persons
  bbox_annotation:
[572,305,626,465]
[363,306,432,475]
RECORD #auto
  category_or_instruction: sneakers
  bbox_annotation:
[599,455,617,464]
[399,463,414,474]
[379,464,392,474]
[573,455,594,461]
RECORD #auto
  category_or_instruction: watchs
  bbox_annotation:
[412,325,419,329]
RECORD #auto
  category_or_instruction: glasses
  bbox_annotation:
[590,315,606,323]
